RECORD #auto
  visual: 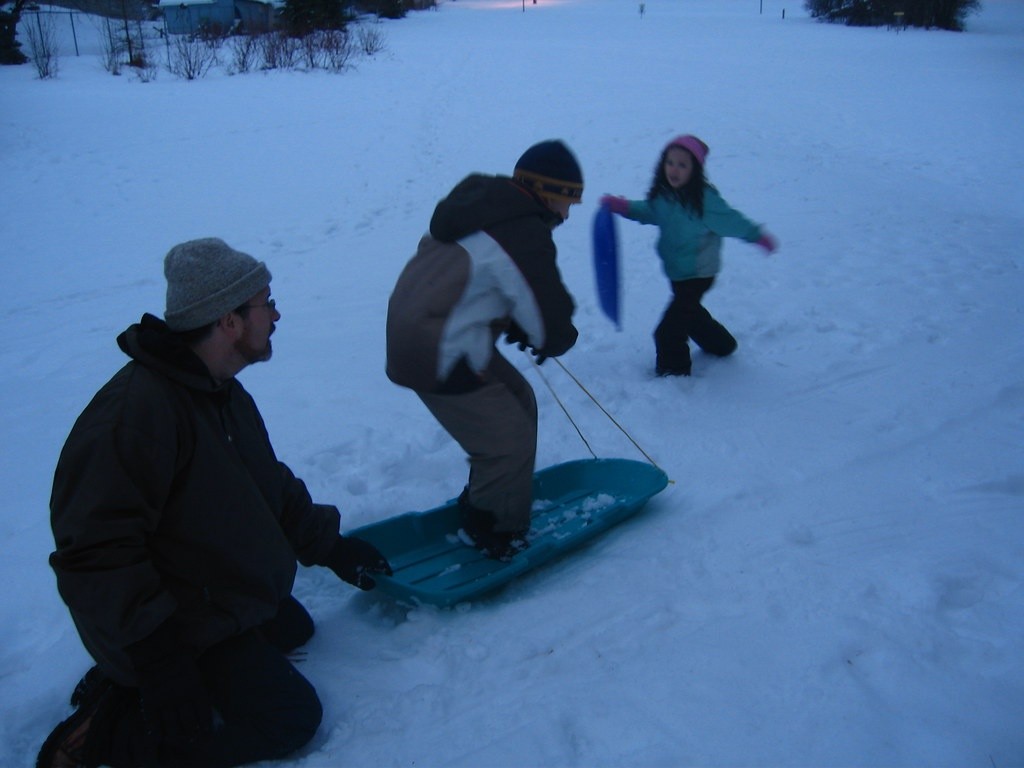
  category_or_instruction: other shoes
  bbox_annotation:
[458,486,531,562]
[35,664,127,768]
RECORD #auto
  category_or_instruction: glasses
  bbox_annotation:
[247,299,275,309]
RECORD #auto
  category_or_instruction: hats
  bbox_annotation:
[512,141,583,203]
[165,238,273,332]
[669,135,709,165]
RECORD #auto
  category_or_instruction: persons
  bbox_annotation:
[599,135,780,375]
[35,236,393,768]
[385,139,585,560]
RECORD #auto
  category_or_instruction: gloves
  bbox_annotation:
[602,196,629,216]
[757,235,775,252]
